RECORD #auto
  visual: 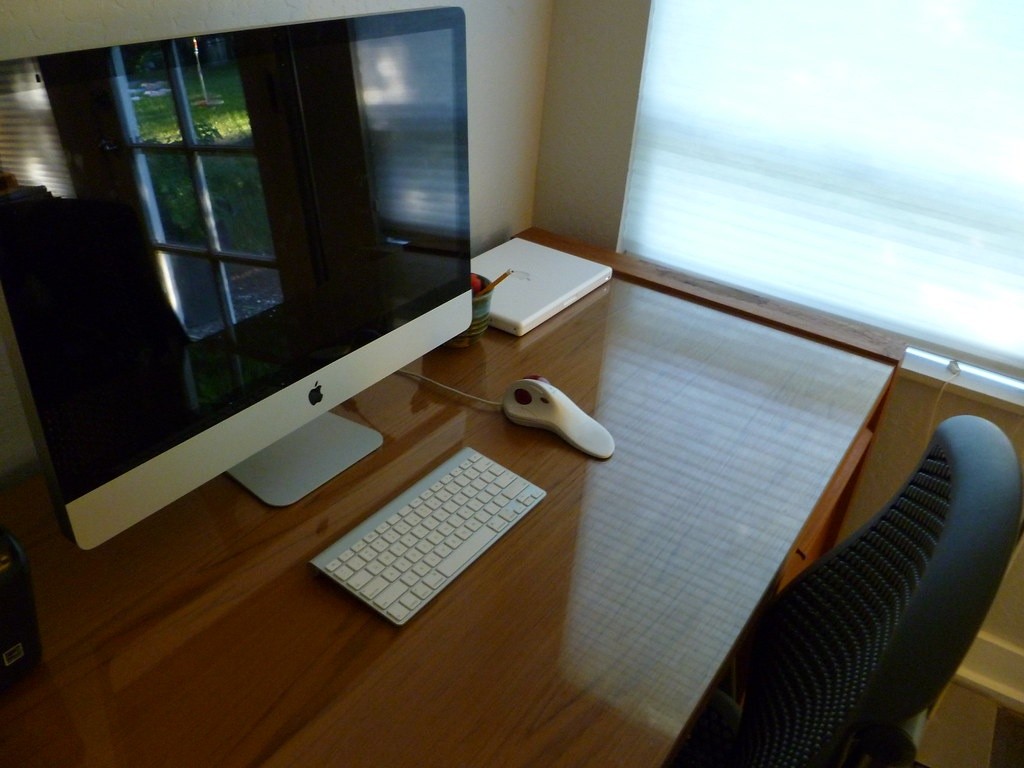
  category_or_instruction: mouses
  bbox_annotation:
[504,375,615,460]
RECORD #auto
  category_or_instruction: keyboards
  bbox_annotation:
[308,448,547,628]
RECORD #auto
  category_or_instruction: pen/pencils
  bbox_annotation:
[477,268,514,296]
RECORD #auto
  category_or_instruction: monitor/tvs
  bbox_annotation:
[0,7,472,552]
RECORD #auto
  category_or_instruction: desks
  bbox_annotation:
[0,226,909,768]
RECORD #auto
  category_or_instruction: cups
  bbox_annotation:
[436,273,492,348]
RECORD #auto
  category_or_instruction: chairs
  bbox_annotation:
[660,414,1023,768]
